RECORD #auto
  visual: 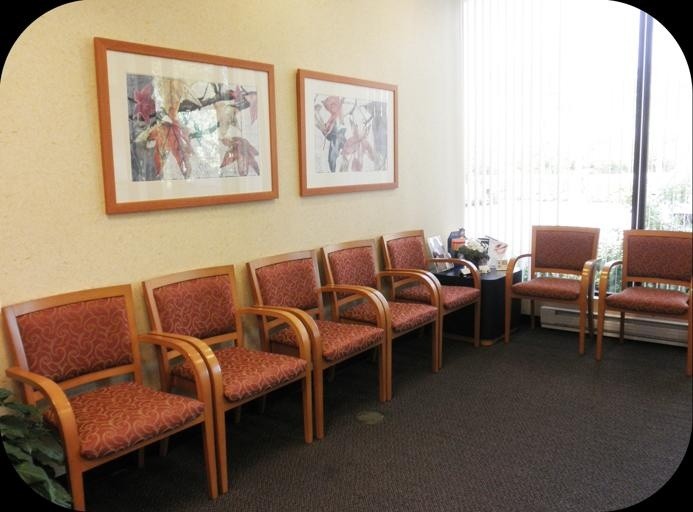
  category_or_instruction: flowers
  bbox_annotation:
[460,236,489,257]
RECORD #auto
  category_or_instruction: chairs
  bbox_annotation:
[141,263,314,494]
[380,231,480,375]
[504,224,693,374]
[243,249,388,442]
[2,283,217,510]
[319,239,440,405]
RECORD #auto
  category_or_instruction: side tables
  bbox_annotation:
[437,258,523,343]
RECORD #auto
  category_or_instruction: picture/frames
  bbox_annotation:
[92,34,281,214]
[293,68,400,197]
